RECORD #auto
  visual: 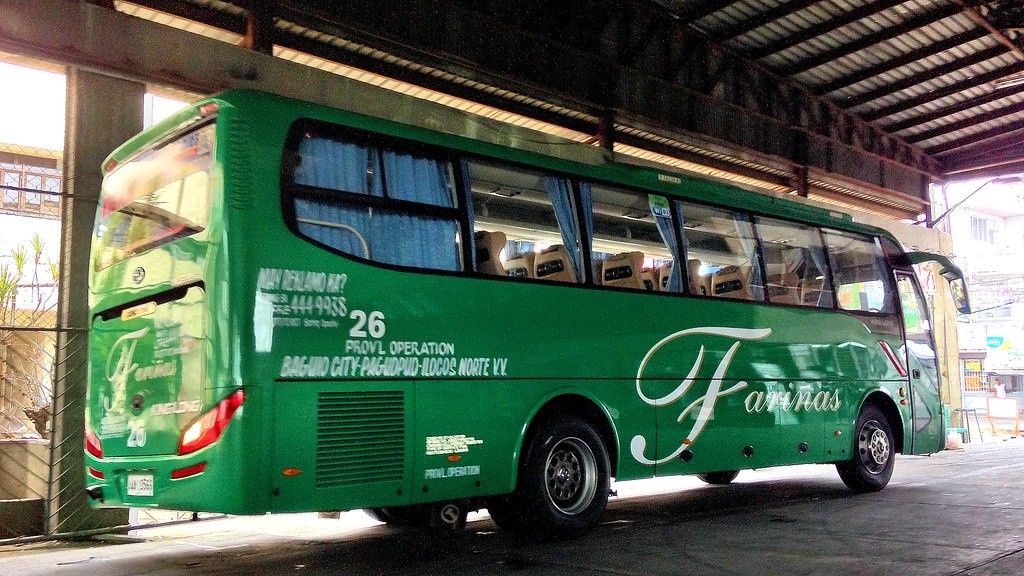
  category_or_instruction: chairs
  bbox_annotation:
[475,231,846,309]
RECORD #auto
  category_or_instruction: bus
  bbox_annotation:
[80,85,972,544]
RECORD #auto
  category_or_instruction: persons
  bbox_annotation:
[993,378,1010,399]
